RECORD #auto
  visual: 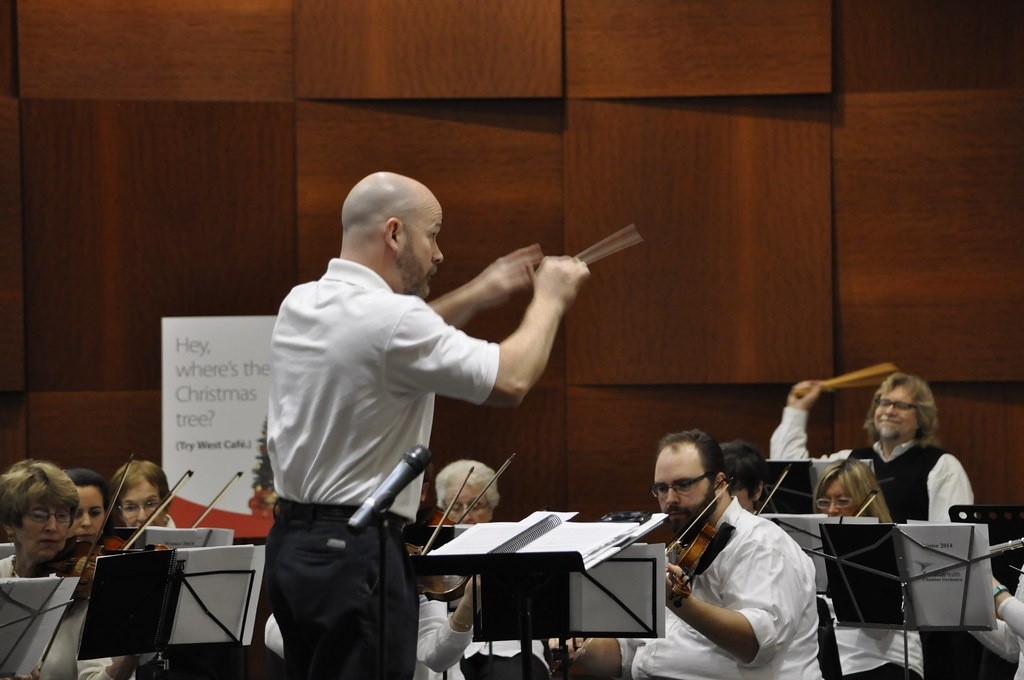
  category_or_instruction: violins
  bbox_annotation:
[101,534,170,551]
[403,541,471,602]
[430,509,455,526]
[35,536,172,586]
[666,522,718,608]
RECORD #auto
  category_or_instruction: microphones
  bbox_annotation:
[347,444,433,535]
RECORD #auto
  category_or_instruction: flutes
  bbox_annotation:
[989,537,1024,555]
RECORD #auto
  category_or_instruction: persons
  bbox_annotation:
[565,430,823,680]
[263,459,480,680]
[264,174,587,680]
[720,440,766,511]
[0,459,176,680]
[770,372,974,525]
[969,550,1024,680]
[436,461,549,680]
[814,458,924,680]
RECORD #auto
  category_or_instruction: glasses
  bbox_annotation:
[816,497,853,511]
[650,472,711,497]
[24,509,71,523]
[122,500,161,514]
[878,397,917,415]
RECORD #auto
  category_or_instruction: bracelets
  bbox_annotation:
[993,584,1009,597]
[452,615,472,628]
